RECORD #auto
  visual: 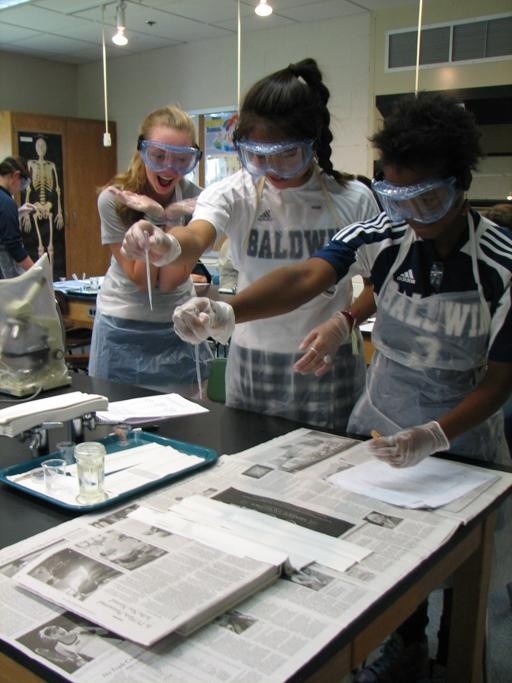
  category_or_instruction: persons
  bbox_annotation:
[87,105,211,402]
[39,625,123,668]
[366,513,402,530]
[0,156,35,279]
[171,91,512,683]
[118,57,386,432]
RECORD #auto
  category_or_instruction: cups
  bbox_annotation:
[73,440,106,497]
[58,277,65,285]
[55,440,76,465]
[41,458,66,492]
[89,276,98,290]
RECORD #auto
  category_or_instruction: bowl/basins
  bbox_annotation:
[194,282,211,296]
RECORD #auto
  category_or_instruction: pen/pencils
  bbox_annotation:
[108,424,160,437]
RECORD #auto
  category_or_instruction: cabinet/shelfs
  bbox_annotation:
[370,0,512,96]
[0,111,118,280]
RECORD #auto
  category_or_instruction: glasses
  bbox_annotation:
[236,138,314,180]
[370,176,455,225]
[139,139,202,176]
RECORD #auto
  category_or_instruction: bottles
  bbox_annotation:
[133,427,142,444]
[117,425,130,446]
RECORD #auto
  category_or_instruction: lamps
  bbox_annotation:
[112,0,129,45]
[101,5,112,147]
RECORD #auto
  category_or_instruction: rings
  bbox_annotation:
[310,347,318,355]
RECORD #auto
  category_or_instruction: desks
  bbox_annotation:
[0,371,512,683]
[52,276,376,366]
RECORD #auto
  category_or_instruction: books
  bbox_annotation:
[172,564,287,639]
[0,390,109,438]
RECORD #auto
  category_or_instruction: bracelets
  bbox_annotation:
[340,310,357,322]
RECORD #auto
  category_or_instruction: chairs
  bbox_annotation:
[65,327,92,376]
